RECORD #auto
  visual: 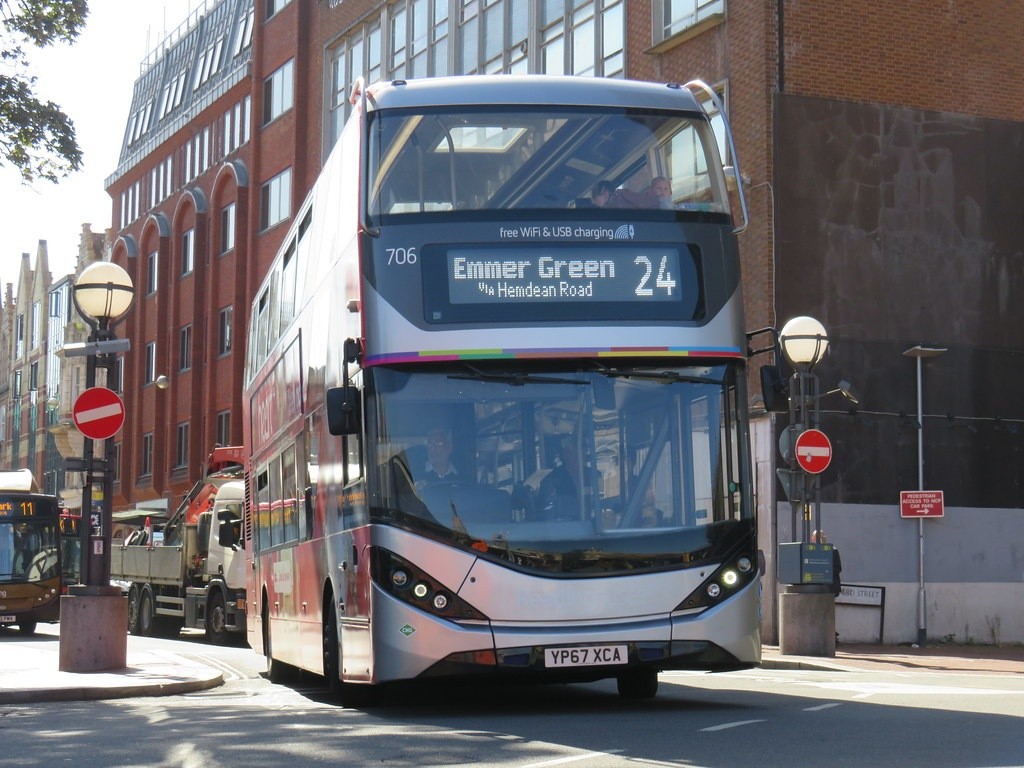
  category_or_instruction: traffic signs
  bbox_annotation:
[900,490,946,517]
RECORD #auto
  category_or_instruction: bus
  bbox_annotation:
[240,75,795,701]
[0,469,72,637]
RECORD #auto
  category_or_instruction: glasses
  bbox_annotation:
[424,441,449,448]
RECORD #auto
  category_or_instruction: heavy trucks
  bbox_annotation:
[109,480,250,647]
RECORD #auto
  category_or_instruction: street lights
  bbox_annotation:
[903,345,949,646]
[777,316,835,593]
[67,260,125,598]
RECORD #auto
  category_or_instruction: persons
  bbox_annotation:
[580,180,615,208]
[651,175,672,198]
[413,429,463,492]
[233,523,240,536]
[537,435,604,521]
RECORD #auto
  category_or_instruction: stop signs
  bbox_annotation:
[795,429,834,475]
[73,386,126,442]
